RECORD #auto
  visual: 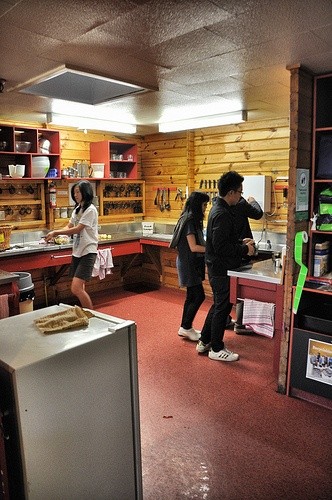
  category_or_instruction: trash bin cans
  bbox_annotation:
[10,271,35,315]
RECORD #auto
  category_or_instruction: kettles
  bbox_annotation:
[77,163,93,178]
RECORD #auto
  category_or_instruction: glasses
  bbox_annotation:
[202,203,208,206]
[230,189,244,196]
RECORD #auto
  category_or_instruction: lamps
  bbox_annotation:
[159,111,247,133]
[47,112,137,133]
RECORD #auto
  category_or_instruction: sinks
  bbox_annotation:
[18,244,38,250]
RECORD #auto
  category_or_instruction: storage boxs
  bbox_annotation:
[314,244,328,277]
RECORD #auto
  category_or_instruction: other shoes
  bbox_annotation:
[233,322,254,334]
[226,320,237,329]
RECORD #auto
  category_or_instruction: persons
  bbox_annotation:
[223,196,263,329]
[44,181,99,310]
[196,171,256,361]
[168,191,212,341]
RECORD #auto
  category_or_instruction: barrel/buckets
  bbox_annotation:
[31,157,50,179]
[90,162,105,178]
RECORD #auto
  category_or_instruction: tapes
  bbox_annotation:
[295,230,310,244]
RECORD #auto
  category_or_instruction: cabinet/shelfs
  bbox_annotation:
[0,124,145,227]
[286,74,332,412]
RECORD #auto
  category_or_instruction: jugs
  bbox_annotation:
[8,164,25,178]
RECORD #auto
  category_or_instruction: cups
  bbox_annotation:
[26,184,35,194]
[117,154,123,160]
[110,154,117,160]
[111,170,127,178]
[127,155,133,161]
[9,184,17,195]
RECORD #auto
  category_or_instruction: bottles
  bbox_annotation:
[62,167,70,177]
[53,208,60,219]
[67,207,74,218]
[60,208,69,219]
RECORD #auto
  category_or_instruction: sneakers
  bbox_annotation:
[196,339,211,353]
[177,326,201,341]
[208,347,240,361]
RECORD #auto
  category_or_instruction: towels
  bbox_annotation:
[90,249,115,281]
[0,294,10,318]
[242,297,275,339]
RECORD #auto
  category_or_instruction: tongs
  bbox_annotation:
[154,189,160,206]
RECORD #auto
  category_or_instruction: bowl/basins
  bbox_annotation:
[38,138,51,154]
[16,141,33,153]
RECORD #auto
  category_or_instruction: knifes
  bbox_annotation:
[200,179,220,204]
